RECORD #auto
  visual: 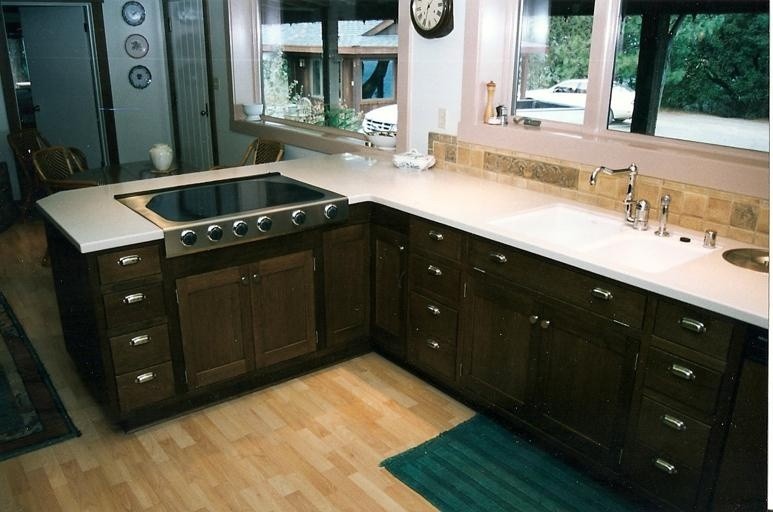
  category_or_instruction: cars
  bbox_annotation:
[516,77,637,125]
[361,98,397,151]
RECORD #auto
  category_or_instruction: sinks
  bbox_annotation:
[474,204,722,279]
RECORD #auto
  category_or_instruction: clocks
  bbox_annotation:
[410,0,454,36]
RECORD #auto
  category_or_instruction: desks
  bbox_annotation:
[64,160,206,186]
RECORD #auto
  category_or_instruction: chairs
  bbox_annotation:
[209,137,286,171]
[31,146,101,266]
[6,127,88,225]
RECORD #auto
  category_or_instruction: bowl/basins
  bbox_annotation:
[361,130,398,150]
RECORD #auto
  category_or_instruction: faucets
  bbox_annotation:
[588,163,637,225]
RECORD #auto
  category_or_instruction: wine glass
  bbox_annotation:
[243,103,263,121]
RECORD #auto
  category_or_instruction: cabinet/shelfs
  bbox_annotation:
[42,216,768,511]
[0,161,19,233]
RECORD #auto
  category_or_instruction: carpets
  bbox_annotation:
[0,293,81,464]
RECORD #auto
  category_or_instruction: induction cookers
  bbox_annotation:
[113,172,349,259]
